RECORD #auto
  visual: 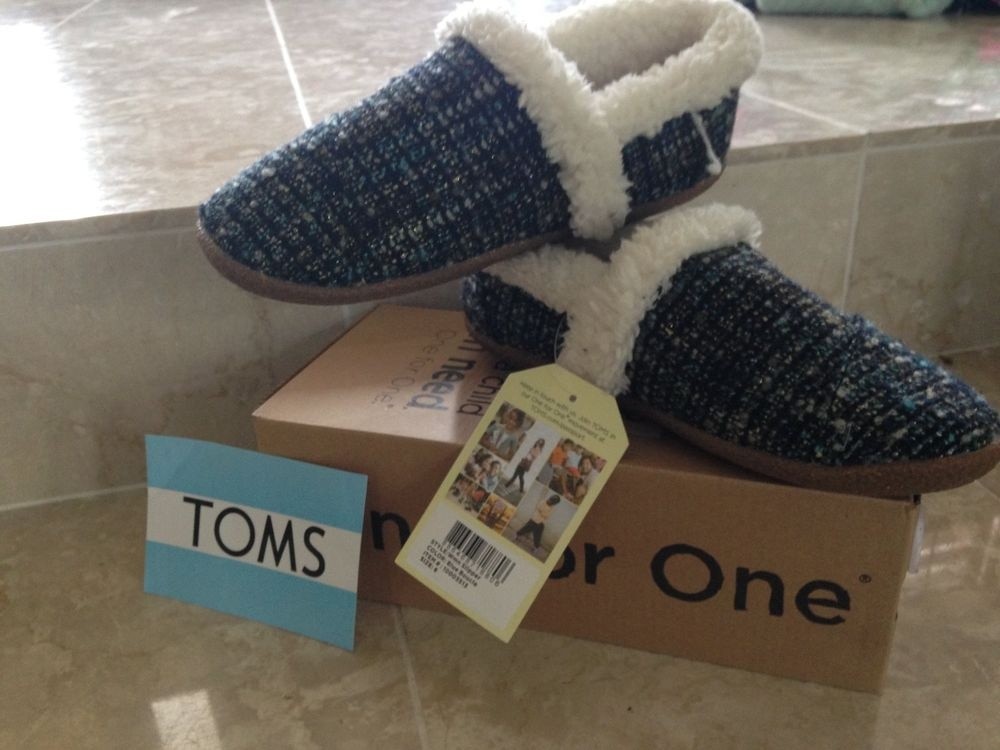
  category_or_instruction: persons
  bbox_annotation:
[451,409,601,557]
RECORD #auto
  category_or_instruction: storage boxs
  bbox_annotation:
[251,303,924,695]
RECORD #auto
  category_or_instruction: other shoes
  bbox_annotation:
[196,0,763,306]
[456,202,999,498]
